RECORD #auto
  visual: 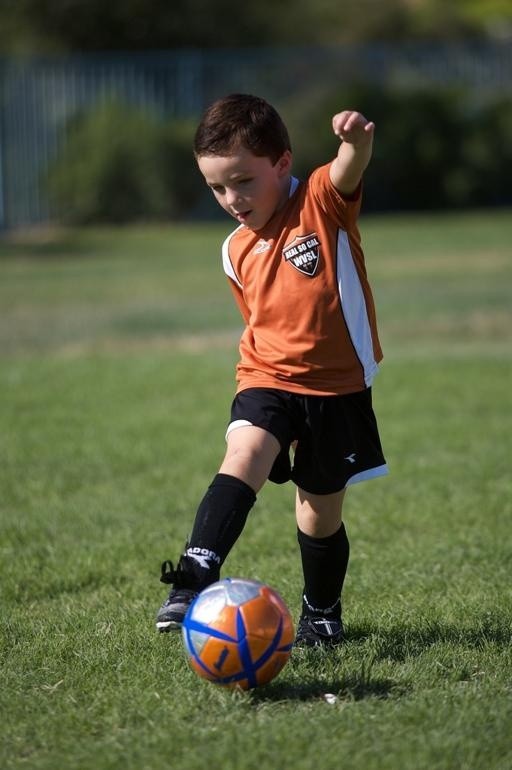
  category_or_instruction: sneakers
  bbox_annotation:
[155,550,218,633]
[293,605,345,647]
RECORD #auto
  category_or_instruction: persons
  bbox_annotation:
[151,92,392,649]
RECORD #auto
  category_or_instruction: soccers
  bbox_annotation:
[182,577,293,692]
